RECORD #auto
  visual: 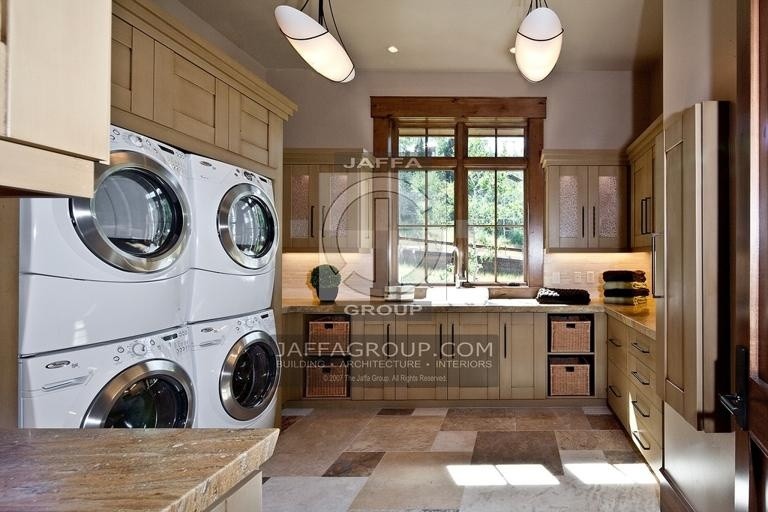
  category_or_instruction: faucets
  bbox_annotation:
[451,247,460,288]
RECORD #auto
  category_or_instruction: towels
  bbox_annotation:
[536,287,591,304]
[603,270,649,306]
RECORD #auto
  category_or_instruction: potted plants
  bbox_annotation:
[310,264,341,301]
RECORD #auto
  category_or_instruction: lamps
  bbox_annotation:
[273,0,357,83]
[515,1,564,83]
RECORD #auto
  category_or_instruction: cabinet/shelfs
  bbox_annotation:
[608,315,629,431]
[654,101,733,433]
[545,165,629,250]
[547,313,596,399]
[630,145,656,249]
[303,313,351,400]
[395,313,500,400]
[350,313,395,400]
[283,165,373,249]
[499,312,548,400]
[629,327,664,482]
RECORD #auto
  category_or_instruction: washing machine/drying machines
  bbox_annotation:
[8,326,197,428]
[189,152,281,321]
[191,309,283,445]
[20,127,197,356]
